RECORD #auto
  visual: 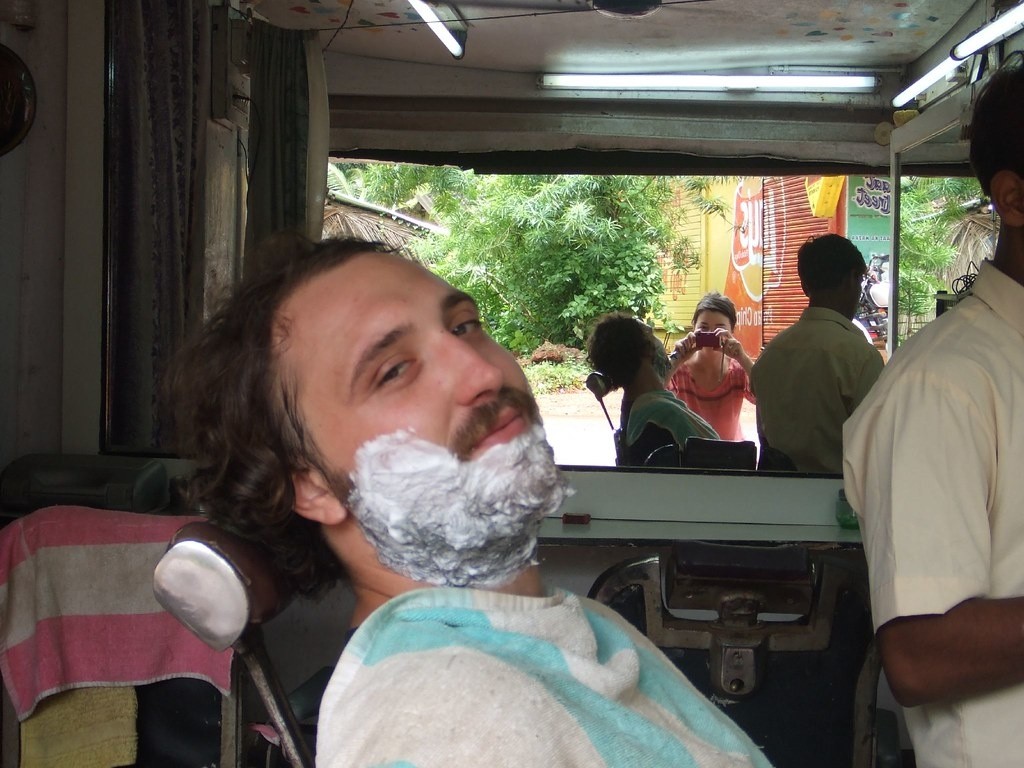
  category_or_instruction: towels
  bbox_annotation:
[0,504,236,768]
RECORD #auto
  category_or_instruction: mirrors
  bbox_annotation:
[320,156,892,479]
[887,76,997,359]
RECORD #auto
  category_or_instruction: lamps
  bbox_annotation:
[537,72,883,95]
[408,0,468,60]
[890,57,970,109]
[949,0,1024,61]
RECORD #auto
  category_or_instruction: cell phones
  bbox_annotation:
[695,332,723,347]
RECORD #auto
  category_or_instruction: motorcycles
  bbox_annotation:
[859,251,890,343]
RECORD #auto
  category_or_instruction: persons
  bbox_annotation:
[842,48,1024,768]
[154,236,778,768]
[666,293,756,441]
[583,311,720,468]
[749,234,885,475]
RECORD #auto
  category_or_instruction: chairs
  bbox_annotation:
[586,373,682,467]
[150,521,315,768]
[585,539,902,768]
